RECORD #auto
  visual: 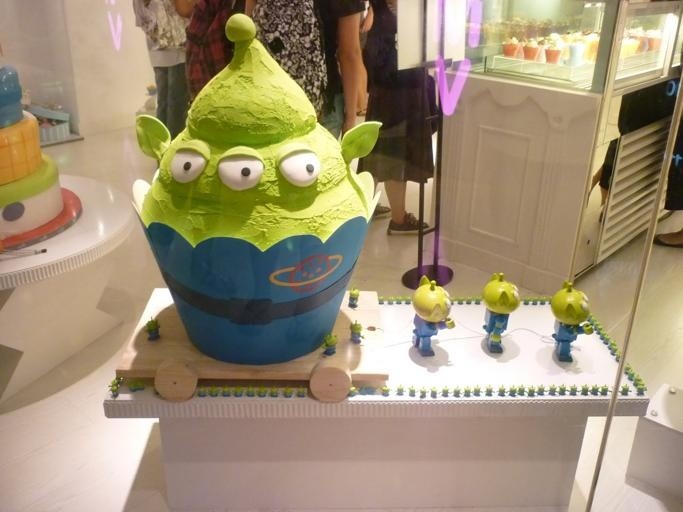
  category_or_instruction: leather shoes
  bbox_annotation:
[654,229,683,248]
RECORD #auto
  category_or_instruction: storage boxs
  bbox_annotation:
[29,105,70,142]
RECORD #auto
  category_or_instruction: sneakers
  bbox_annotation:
[387,213,431,235]
[374,206,392,219]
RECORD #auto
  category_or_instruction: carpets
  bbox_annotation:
[40,133,84,147]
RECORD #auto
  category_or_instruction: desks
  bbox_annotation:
[104,289,650,512]
[0,174,136,292]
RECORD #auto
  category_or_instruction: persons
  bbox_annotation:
[656,133,683,247]
[133,0,428,236]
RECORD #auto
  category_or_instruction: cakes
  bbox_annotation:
[0,62,63,241]
[501,29,654,66]
[135,13,383,365]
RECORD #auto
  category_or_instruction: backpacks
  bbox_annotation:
[186,1,328,124]
